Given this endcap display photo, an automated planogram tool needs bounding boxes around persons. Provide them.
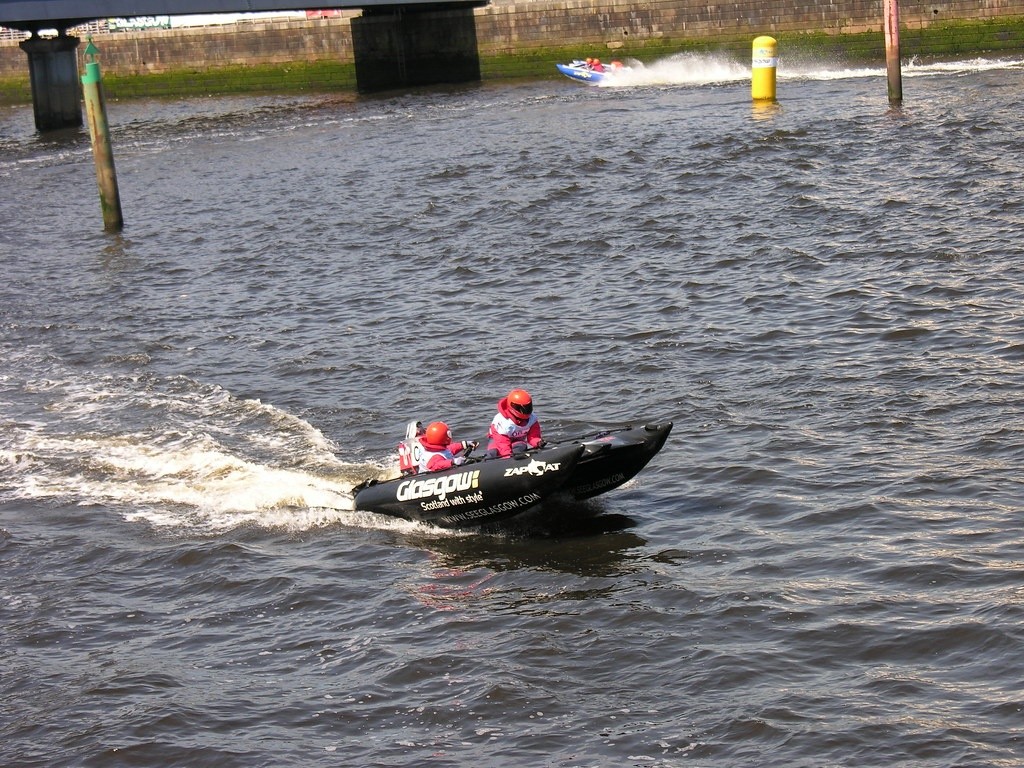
[487,389,544,460]
[585,58,605,72]
[418,421,479,474]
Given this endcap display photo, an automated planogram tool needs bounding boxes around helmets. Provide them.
[508,390,532,419]
[426,422,452,445]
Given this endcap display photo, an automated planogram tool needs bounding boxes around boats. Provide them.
[556,59,632,87]
[350,422,674,529]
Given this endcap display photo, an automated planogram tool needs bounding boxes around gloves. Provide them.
[538,440,547,448]
[454,457,467,465]
[461,440,479,451]
